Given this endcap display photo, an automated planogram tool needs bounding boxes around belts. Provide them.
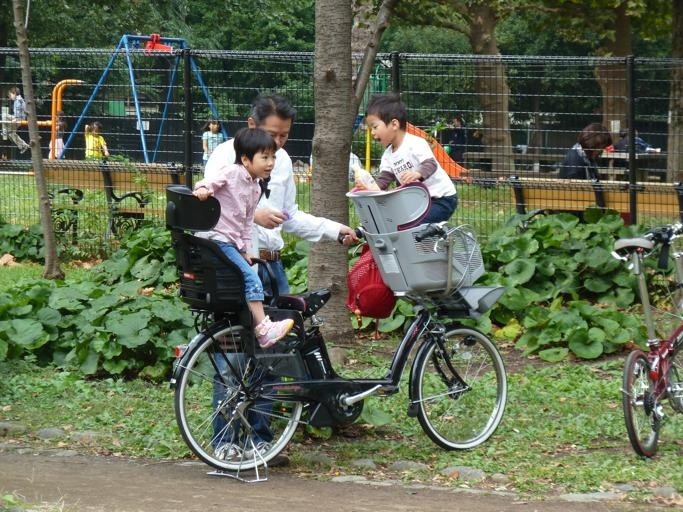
[241,249,280,261]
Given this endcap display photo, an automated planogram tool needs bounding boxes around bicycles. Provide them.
[613,183,683,457]
[166,182,507,482]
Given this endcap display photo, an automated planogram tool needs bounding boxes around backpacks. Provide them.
[346,244,399,319]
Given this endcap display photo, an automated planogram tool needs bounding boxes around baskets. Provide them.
[381,228,485,293]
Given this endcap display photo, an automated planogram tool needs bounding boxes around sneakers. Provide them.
[253,315,294,349]
[210,443,290,468]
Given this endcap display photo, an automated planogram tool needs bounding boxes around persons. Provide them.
[6,87,29,155]
[190,128,295,350]
[559,122,613,181]
[351,93,458,229]
[613,127,631,170]
[200,115,225,165]
[84,122,110,162]
[47,111,68,160]
[635,130,661,168]
[205,92,359,470]
[449,115,480,162]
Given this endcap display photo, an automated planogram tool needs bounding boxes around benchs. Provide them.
[511,176,683,229]
[33,158,186,246]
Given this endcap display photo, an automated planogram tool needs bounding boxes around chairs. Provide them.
[165,184,282,314]
[102,160,152,241]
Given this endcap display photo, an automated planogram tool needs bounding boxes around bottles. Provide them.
[351,162,382,192]
